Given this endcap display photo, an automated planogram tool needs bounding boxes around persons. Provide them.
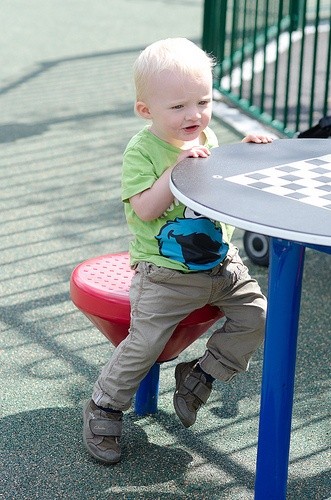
[82,37,273,461]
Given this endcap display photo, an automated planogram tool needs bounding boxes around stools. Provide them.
[71,253,225,414]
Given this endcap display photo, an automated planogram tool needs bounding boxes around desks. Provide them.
[168,138,331,500]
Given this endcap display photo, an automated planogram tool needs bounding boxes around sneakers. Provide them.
[174,361,211,428]
[83,398,123,462]
